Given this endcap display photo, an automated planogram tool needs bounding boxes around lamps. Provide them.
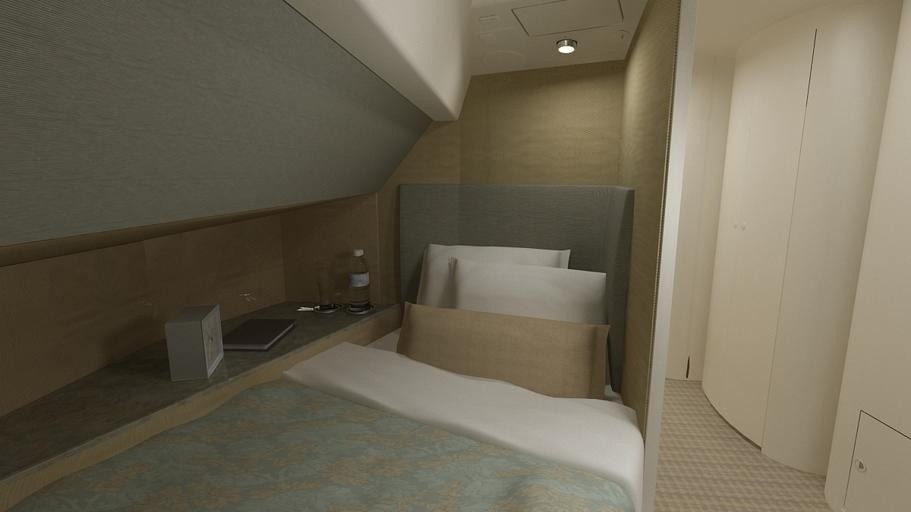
[556,36,581,59]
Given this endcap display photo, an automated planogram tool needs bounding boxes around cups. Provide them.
[314,257,337,310]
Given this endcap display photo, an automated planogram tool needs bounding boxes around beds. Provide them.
[6,244,642,512]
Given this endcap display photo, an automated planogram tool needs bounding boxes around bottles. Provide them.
[348,247,370,313]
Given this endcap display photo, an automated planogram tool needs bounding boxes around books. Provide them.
[221,316,298,353]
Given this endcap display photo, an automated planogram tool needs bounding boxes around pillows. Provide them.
[393,244,614,399]
